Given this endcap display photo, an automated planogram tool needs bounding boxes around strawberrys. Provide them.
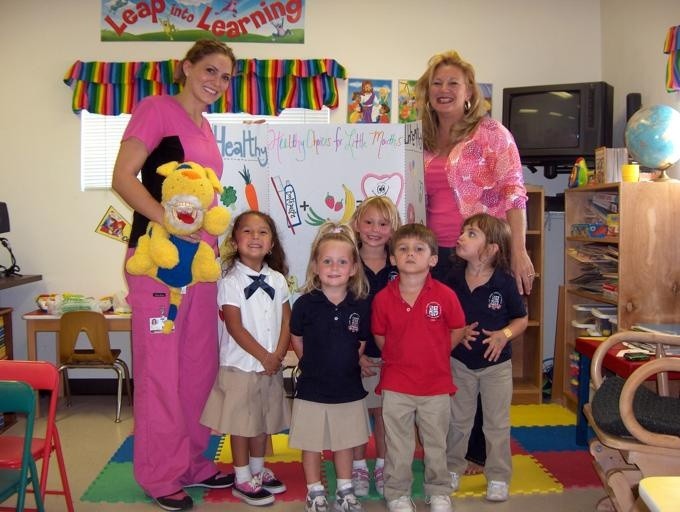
[325,192,334,209]
[335,199,343,211]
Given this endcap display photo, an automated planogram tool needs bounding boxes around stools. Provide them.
[583,326,679,512]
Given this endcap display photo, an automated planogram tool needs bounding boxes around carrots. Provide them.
[238,164,259,212]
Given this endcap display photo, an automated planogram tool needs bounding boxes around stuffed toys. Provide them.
[126,160,232,334]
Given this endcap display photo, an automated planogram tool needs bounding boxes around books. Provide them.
[567,146,679,402]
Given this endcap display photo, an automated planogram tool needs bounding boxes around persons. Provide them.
[409,51,537,476]
[434,213,529,501]
[112,38,237,512]
[198,209,293,507]
[290,222,372,512]
[369,223,467,511]
[344,195,401,499]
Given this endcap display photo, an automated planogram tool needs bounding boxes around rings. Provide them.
[528,273,533,277]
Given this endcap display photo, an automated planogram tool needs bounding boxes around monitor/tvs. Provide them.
[502,81,614,165]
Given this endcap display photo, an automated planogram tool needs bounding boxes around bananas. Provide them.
[339,184,356,225]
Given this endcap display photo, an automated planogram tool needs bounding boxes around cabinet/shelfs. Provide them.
[508,186,544,407]
[0,306,13,360]
[562,179,679,418]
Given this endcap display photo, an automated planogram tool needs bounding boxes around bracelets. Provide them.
[501,327,513,342]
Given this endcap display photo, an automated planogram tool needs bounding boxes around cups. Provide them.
[621,164,639,183]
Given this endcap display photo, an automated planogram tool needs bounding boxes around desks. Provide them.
[0,272,43,292]
[22,308,134,417]
[573,335,679,448]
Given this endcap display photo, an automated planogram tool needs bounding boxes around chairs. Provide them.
[0,358,75,512]
[49,310,133,424]
[0,380,46,512]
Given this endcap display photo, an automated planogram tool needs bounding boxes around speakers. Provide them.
[627,93,642,122]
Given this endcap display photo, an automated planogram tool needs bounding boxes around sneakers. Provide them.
[374,465,384,496]
[487,481,508,501]
[232,476,275,505]
[184,471,234,488]
[386,496,413,512]
[306,485,329,512]
[252,471,286,493]
[352,467,369,497]
[335,483,368,512]
[144,489,193,511]
[430,496,453,512]
[449,471,459,492]
